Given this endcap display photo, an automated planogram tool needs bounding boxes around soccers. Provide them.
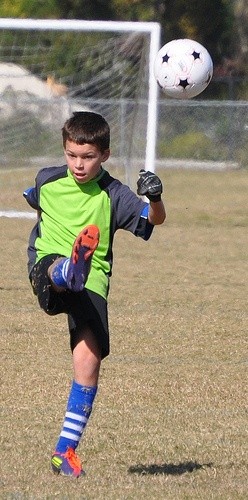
[152,37,214,102]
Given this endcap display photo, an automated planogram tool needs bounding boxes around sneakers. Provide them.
[67,224,100,291]
[51,444,87,476]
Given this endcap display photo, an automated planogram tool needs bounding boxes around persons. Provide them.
[23,111,165,475]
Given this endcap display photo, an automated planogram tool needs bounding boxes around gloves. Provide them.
[137,168,162,202]
